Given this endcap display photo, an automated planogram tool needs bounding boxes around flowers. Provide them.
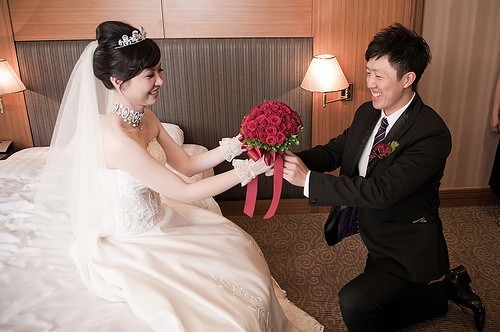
[239,100,304,152]
[368,141,400,159]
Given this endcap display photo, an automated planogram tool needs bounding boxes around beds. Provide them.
[0,147,223,332]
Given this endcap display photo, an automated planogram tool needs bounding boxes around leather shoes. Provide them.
[446,265,484,332]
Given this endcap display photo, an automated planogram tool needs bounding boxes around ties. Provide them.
[355,117,388,232]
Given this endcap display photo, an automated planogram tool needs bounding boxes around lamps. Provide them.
[0,58,27,115]
[299,53,353,108]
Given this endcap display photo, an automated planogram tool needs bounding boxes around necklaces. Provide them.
[110,103,146,130]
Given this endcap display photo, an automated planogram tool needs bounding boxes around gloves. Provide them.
[218,133,247,162]
[232,154,275,187]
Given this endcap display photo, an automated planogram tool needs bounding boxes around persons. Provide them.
[265,24,485,332]
[0,21,324,332]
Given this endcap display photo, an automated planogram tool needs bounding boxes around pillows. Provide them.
[162,122,185,149]
